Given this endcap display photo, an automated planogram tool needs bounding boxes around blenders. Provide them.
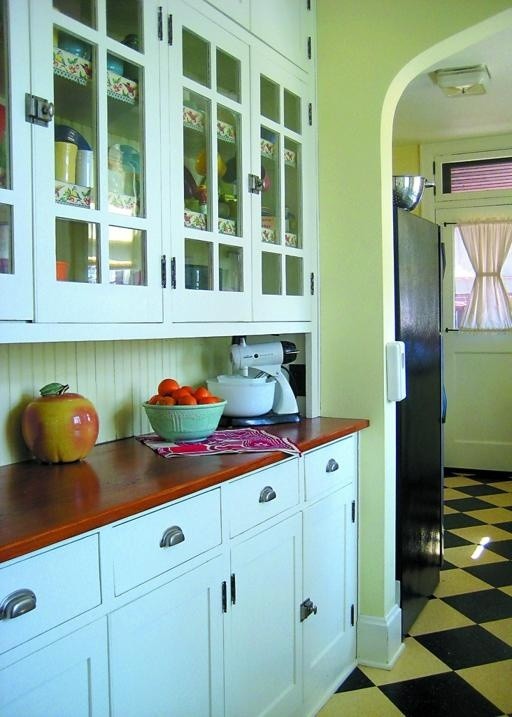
[205,337,302,428]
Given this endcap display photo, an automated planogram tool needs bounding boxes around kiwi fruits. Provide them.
[218,201,231,218]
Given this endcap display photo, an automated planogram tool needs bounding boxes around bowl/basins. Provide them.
[392,176,426,212]
[141,397,228,442]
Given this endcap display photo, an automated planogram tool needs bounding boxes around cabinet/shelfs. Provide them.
[164,2,320,340]
[0,1,164,344]
[1,480,229,715]
[221,430,363,715]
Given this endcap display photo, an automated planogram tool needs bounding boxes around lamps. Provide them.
[426,65,494,101]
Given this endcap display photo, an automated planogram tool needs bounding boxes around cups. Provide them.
[75,149,95,189]
[194,147,272,192]
[54,141,79,186]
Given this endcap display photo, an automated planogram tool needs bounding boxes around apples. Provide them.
[20,383,100,463]
[5,394,34,461]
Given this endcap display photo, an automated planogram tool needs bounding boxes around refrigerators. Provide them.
[390,207,448,643]
